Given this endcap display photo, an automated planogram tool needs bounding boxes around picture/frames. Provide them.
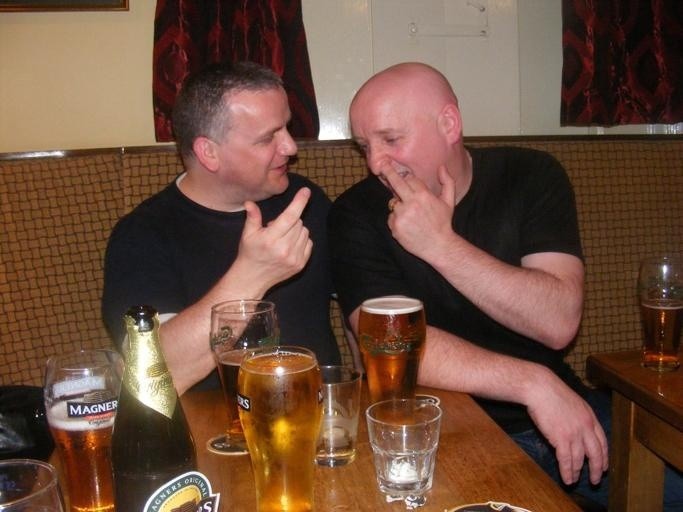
[0,0,130,14]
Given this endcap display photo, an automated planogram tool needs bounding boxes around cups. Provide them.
[43,349,118,511]
[312,366,360,465]
[358,296,427,427]
[238,345,323,511]
[365,397,441,499]
[0,458,65,511]
[635,256,682,373]
[209,300,280,441]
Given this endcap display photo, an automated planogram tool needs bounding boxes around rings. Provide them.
[391,200,399,211]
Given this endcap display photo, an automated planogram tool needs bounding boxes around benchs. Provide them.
[0,126,681,510]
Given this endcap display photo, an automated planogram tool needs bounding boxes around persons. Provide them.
[100,60,365,397]
[326,61,683,512]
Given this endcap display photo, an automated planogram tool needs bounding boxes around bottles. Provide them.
[108,307,199,511]
